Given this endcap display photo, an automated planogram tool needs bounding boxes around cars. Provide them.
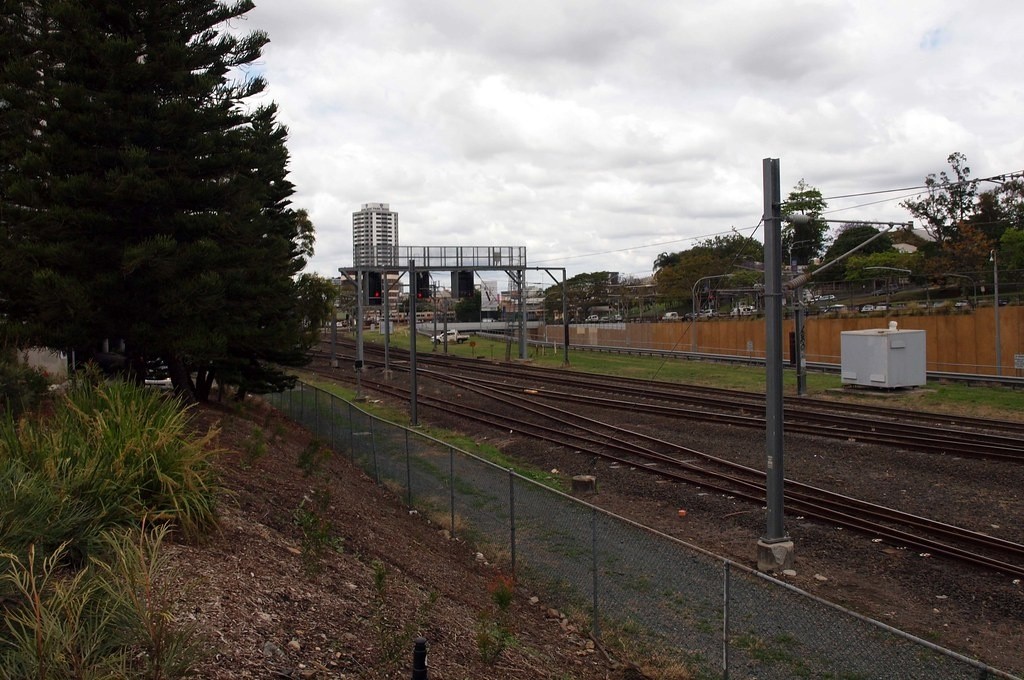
[955,299,973,309]
[570,314,623,323]
[662,306,757,320]
[860,301,944,312]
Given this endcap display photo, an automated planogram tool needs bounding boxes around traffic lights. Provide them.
[369,271,381,305]
[417,270,429,298]
[459,272,473,297]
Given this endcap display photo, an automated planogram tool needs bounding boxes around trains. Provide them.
[381,309,564,323]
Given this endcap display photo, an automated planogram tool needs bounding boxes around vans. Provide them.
[824,304,847,313]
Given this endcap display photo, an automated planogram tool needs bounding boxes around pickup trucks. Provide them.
[431,329,470,345]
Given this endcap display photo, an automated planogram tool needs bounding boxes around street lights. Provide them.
[543,292,559,343]
[692,273,734,352]
[990,249,1002,376]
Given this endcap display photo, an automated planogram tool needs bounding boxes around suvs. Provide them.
[872,283,899,297]
